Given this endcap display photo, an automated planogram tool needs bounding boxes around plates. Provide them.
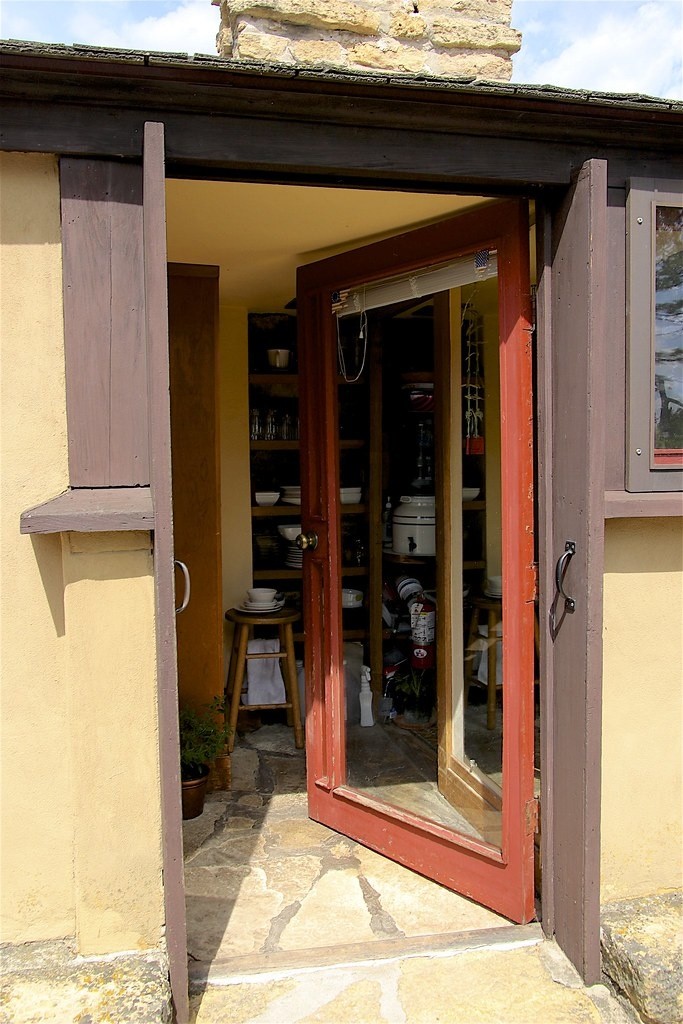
[280,485,301,505]
[484,585,502,599]
[284,543,302,568]
[234,599,281,613]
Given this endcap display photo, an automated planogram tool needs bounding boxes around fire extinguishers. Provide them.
[410,589,438,671]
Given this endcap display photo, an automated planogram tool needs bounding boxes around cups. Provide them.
[267,349,289,368]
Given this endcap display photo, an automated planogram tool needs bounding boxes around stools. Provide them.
[224,609,304,752]
[464,596,540,731]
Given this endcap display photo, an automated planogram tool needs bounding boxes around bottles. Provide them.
[248,405,299,441]
[287,660,305,727]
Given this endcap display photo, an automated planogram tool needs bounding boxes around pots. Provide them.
[393,495,436,556]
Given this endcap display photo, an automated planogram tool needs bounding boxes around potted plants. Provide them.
[179,695,234,821]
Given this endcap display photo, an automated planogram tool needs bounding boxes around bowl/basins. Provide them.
[278,525,301,541]
[339,487,361,504]
[462,487,480,501]
[251,491,280,507]
[487,575,501,588]
[247,588,277,602]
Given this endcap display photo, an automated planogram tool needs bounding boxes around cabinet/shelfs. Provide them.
[248,313,486,724]
